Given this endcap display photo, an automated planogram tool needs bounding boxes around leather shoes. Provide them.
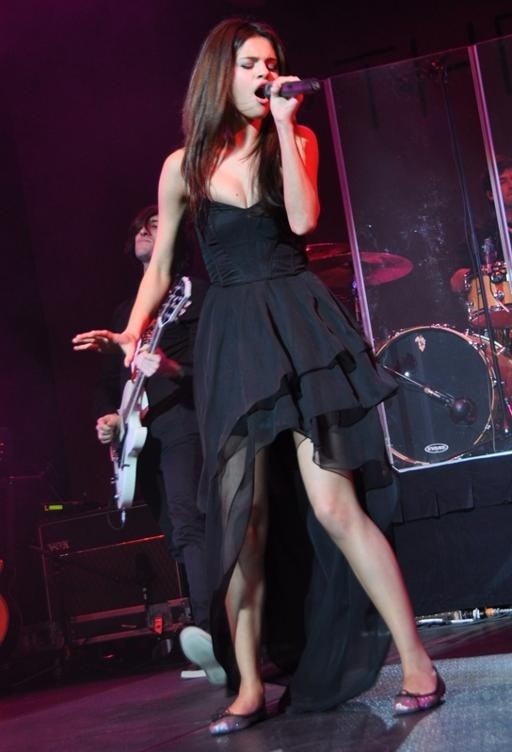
[393,666,445,715]
[209,698,265,734]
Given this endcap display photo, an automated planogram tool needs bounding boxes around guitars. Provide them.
[109,277,192,509]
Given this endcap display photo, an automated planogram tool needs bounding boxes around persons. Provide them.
[69,205,248,693]
[64,15,447,738]
[455,156,512,279]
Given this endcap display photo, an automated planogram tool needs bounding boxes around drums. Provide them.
[461,260,512,331]
[375,325,512,466]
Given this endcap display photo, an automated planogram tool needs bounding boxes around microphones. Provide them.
[447,393,480,425]
[262,75,324,100]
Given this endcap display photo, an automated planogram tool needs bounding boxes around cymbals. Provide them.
[303,249,411,289]
